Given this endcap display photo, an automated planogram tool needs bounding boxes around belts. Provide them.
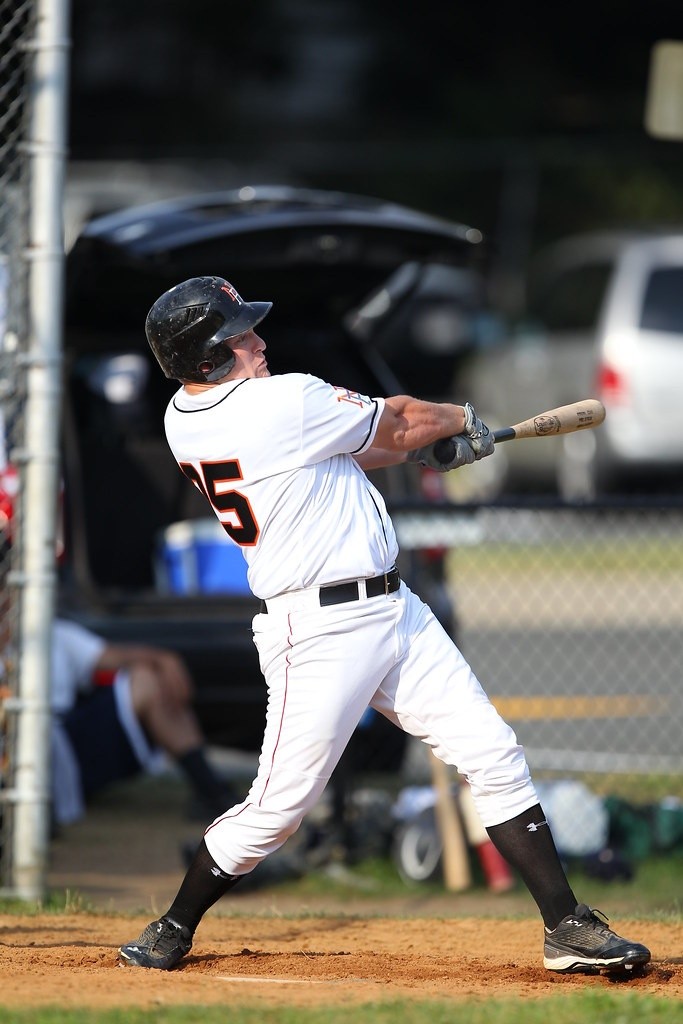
[260,566,401,614]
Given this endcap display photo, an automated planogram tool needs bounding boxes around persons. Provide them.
[118,276,652,973]
[49,620,244,825]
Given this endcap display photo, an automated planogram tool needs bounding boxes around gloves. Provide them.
[458,402,495,461]
[405,434,475,472]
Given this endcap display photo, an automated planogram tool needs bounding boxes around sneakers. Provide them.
[118,917,192,970]
[543,904,651,976]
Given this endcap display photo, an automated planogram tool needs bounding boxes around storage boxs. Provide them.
[161,520,253,594]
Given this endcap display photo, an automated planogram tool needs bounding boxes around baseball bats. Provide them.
[432,398,606,463]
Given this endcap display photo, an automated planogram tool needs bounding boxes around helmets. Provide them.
[144,277,273,383]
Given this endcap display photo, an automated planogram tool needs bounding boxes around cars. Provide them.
[444,232,683,511]
[59,183,484,775]
